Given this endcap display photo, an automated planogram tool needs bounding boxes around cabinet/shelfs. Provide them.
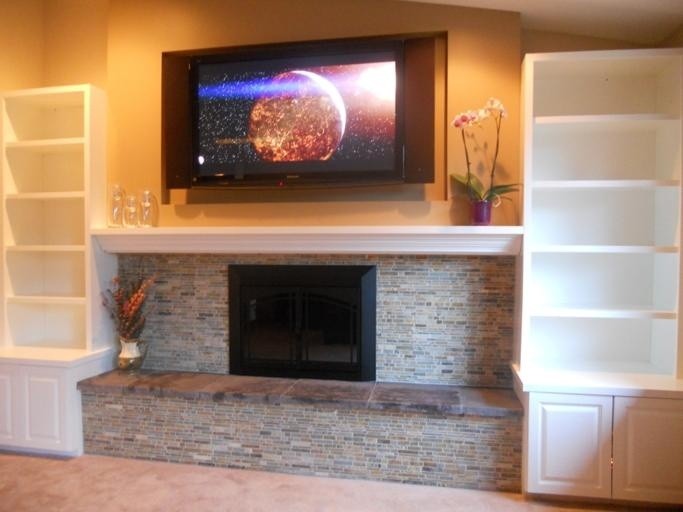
[1,84,111,458]
[507,49,683,506]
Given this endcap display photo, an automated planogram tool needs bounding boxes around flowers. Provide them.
[101,273,160,341]
[448,97,521,205]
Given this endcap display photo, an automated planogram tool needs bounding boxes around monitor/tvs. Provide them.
[186,33,405,189]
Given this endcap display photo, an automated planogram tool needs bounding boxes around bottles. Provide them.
[106,183,158,230]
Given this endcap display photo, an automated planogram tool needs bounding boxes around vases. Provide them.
[470,200,493,226]
[116,336,144,372]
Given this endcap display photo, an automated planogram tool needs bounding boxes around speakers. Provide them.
[404,36,436,185]
[161,49,187,190]
